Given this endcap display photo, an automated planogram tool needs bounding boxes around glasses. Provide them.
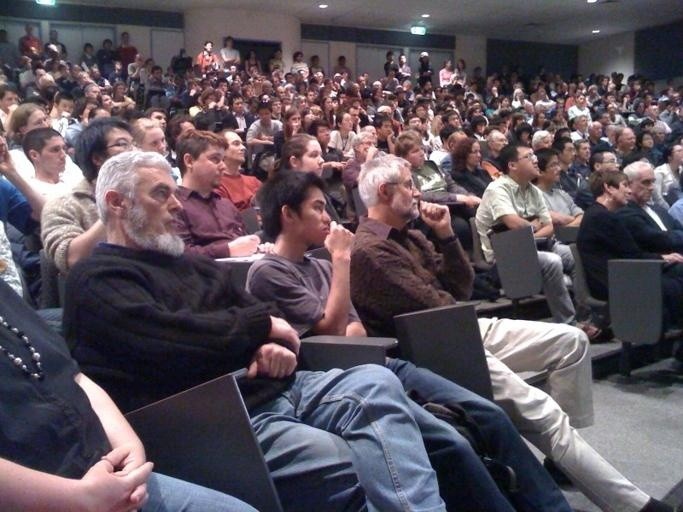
[103,141,138,152]
[387,178,414,191]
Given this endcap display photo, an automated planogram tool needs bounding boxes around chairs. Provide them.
[41,142,683,512]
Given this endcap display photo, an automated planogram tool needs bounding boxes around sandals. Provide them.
[577,322,603,341]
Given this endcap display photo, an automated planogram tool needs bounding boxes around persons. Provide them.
[0,24,683,511]
